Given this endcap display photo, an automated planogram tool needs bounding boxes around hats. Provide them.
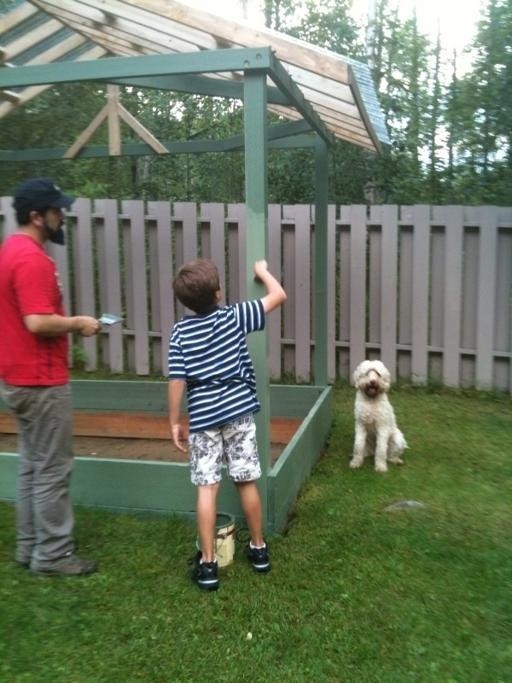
[12,179,75,210]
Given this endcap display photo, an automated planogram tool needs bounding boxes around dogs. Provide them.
[348,359,409,473]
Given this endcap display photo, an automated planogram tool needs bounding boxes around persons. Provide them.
[169,259,289,588]
[1,178,103,575]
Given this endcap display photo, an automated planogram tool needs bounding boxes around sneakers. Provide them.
[189,550,219,592]
[14,544,32,566]
[243,539,271,573]
[30,551,98,578]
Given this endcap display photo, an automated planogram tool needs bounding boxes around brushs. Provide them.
[98,313,125,326]
